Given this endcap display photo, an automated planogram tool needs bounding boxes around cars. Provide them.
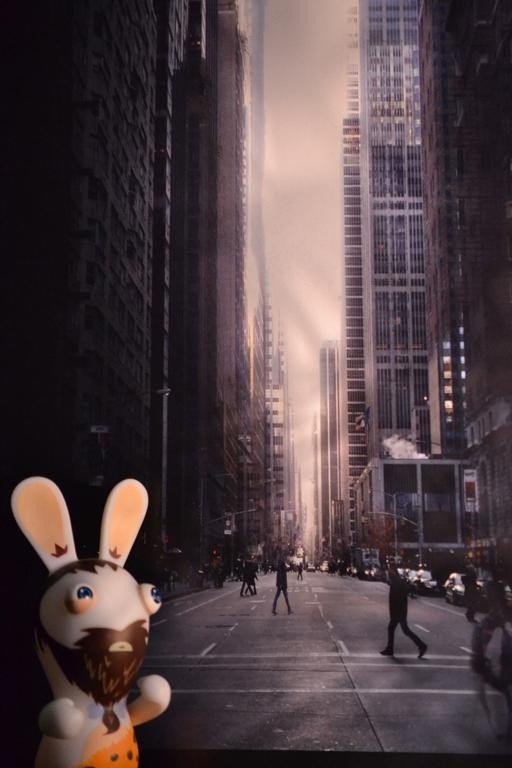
[285,556,512,614]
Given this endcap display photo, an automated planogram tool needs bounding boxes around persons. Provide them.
[240,566,258,595]
[296,561,303,581]
[272,559,292,615]
[461,565,479,624]
[380,568,427,658]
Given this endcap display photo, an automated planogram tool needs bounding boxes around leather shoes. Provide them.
[417,644,428,658]
[379,647,394,655]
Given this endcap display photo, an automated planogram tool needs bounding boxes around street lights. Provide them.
[197,477,278,589]
[362,488,423,570]
